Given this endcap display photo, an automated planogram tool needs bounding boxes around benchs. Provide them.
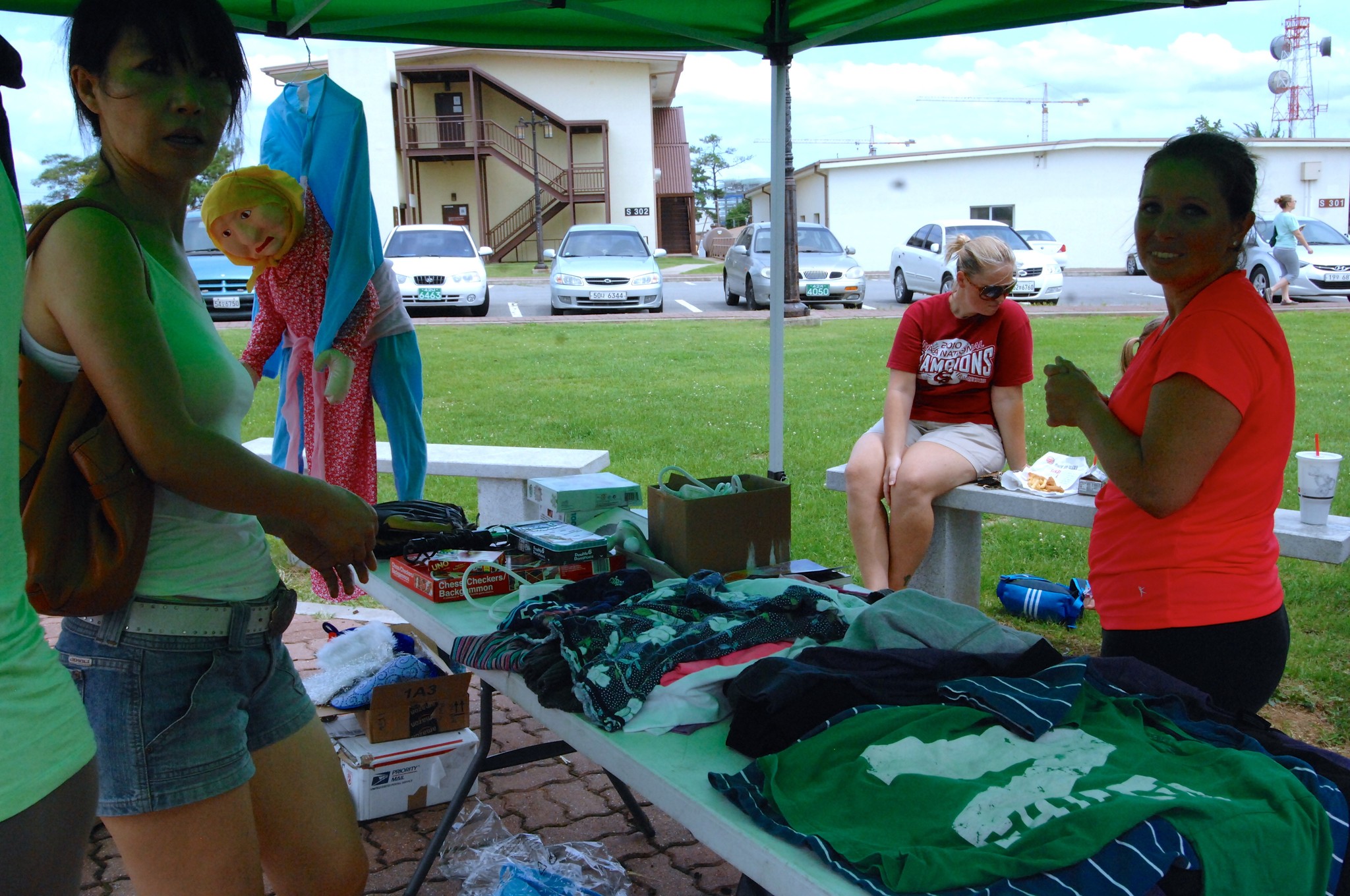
[823,463,1350,610]
[241,437,611,531]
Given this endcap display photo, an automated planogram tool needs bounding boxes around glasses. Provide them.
[962,277,1017,299]
[1287,200,1297,204]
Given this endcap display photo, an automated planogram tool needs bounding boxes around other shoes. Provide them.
[1280,299,1298,306]
[1265,288,1273,305]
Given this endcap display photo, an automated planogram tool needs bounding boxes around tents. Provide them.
[0,0,1231,479]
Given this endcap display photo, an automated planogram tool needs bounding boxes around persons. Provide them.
[0,155,98,896]
[18,0,376,896]
[1045,133,1296,714]
[844,234,1034,592]
[201,165,377,604]
[1120,317,1169,375]
[1264,194,1313,306]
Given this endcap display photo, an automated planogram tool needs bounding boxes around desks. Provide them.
[350,559,873,896]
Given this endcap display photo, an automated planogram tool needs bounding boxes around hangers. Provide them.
[288,37,327,87]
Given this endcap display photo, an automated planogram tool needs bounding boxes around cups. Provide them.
[1295,451,1343,525]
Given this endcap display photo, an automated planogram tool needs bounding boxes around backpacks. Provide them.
[998,572,1089,628]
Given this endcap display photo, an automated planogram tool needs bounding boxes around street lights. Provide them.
[514,110,554,270]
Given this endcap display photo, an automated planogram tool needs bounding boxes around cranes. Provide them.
[750,125,915,156]
[910,83,1090,142]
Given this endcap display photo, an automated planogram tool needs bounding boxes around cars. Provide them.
[181,209,255,322]
[891,219,1067,306]
[543,224,666,316]
[1127,246,1147,276]
[724,221,865,311]
[381,224,495,315]
[1237,214,1350,303]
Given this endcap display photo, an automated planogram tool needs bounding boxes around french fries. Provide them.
[1027,472,1064,493]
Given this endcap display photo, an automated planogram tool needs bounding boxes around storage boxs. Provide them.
[391,545,626,603]
[527,472,643,511]
[509,520,607,564]
[320,712,478,821]
[315,623,474,743]
[540,505,630,525]
[646,475,792,578]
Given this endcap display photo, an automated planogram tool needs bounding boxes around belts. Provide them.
[74,588,297,638]
